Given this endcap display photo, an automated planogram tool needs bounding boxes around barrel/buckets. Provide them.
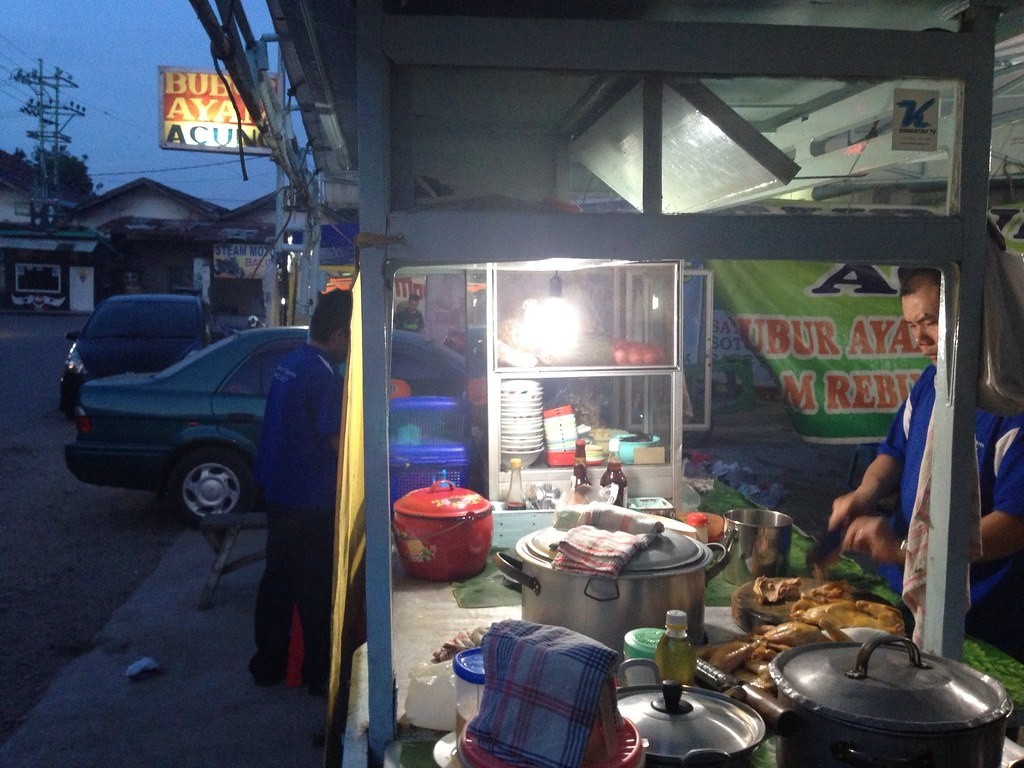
[456,712,646,768]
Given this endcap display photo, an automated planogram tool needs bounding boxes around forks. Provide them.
[527,481,563,510]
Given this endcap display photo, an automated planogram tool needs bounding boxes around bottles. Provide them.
[686,512,709,545]
[571,439,593,504]
[652,609,696,688]
[504,458,526,510]
[599,439,628,509]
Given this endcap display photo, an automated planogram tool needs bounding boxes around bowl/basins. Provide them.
[723,507,794,586]
[452,647,489,714]
[501,379,545,469]
[610,434,661,464]
[543,403,577,466]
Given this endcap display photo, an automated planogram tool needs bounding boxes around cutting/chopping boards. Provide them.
[730,576,854,635]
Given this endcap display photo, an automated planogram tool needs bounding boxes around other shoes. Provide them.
[249,654,287,684]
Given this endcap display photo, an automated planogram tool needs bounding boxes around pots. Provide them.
[492,526,732,661]
[393,480,494,580]
[769,634,1014,768]
[617,656,766,768]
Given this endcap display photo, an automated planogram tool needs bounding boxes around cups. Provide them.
[624,627,666,687]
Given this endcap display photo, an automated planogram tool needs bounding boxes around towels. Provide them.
[466,618,622,768]
[547,498,666,580]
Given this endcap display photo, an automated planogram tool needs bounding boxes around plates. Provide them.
[433,731,462,768]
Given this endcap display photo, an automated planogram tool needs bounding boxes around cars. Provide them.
[59,295,215,421]
[63,324,470,532]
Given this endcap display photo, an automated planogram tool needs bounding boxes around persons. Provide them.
[829,263,1024,643]
[394,293,424,336]
[248,288,353,696]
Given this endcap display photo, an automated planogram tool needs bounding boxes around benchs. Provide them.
[197,513,267,610]
